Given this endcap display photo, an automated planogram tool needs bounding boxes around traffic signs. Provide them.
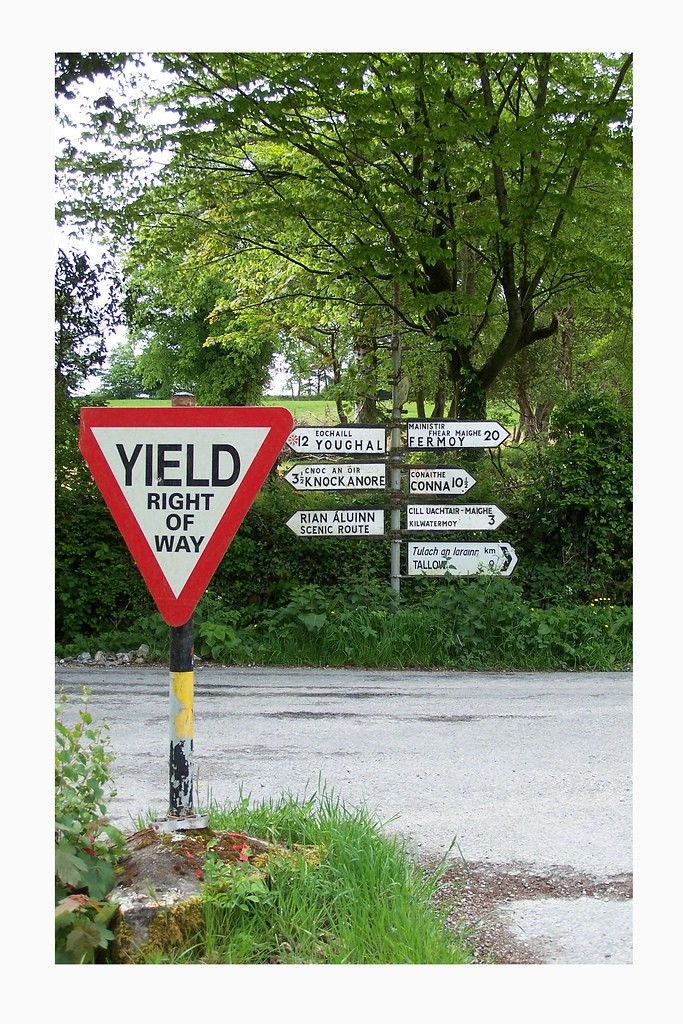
[284,426,388,456]
[408,539,518,578]
[409,465,477,497]
[283,462,386,493]
[407,417,509,449]
[406,503,509,531]
[284,509,386,537]
